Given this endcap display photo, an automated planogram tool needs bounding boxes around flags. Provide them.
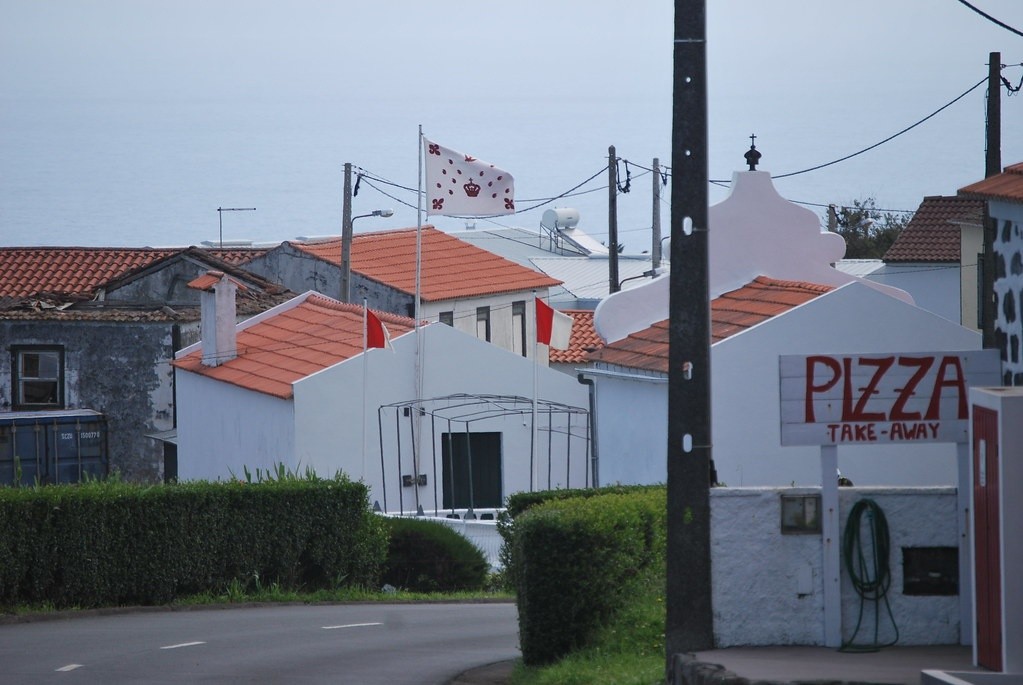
[422,134,517,217]
